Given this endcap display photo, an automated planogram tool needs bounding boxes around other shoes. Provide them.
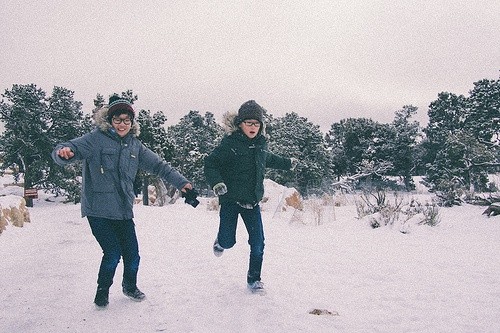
[94,286,109,310]
[247,282,265,294]
[122,286,146,302]
[213,239,224,258]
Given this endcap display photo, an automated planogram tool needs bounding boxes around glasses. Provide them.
[112,115,132,125]
[242,120,261,128]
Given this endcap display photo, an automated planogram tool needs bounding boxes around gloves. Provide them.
[290,158,299,171]
[212,182,228,197]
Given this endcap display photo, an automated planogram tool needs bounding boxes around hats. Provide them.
[108,96,135,118]
[234,100,263,128]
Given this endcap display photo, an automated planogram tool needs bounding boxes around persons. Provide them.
[52,96,193,311]
[204,100,300,295]
